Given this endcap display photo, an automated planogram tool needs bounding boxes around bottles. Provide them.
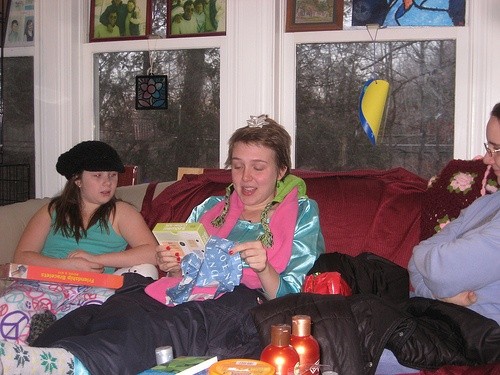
[289,315,321,375]
[260,324,300,375]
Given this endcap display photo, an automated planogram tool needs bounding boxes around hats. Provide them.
[57,141,126,179]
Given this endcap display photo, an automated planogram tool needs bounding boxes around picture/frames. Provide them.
[89,0,151,43]
[285,0,344,32]
[166,0,226,39]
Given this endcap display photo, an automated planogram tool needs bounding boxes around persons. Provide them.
[0,140,160,345]
[374,102,500,375]
[23,20,35,41]
[171,0,213,34]
[8,20,21,43]
[0,114,326,375]
[95,0,143,38]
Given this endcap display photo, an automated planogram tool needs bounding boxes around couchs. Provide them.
[0,158,500,375]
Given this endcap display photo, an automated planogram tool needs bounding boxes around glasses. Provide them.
[484,143,500,156]
